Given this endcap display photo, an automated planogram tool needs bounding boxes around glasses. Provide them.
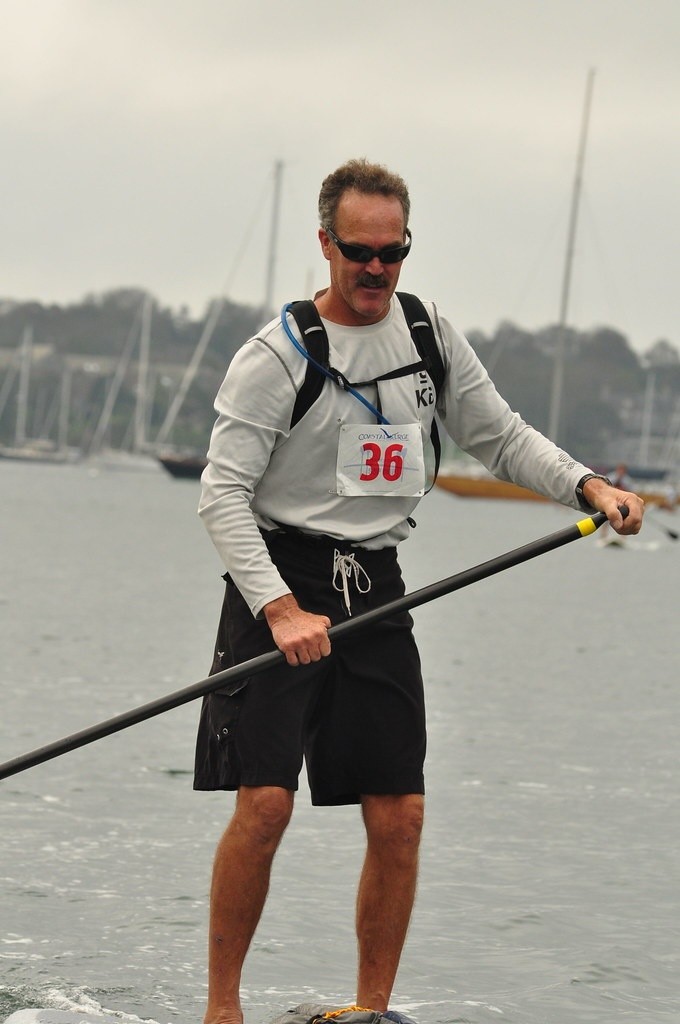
[321,223,412,264]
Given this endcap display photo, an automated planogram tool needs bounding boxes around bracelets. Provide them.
[576,474,611,514]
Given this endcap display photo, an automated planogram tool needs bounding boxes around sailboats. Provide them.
[430,70,679,509]
[0,326,70,464]
[155,158,283,481]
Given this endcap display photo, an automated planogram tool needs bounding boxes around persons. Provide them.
[193,158,644,1024]
